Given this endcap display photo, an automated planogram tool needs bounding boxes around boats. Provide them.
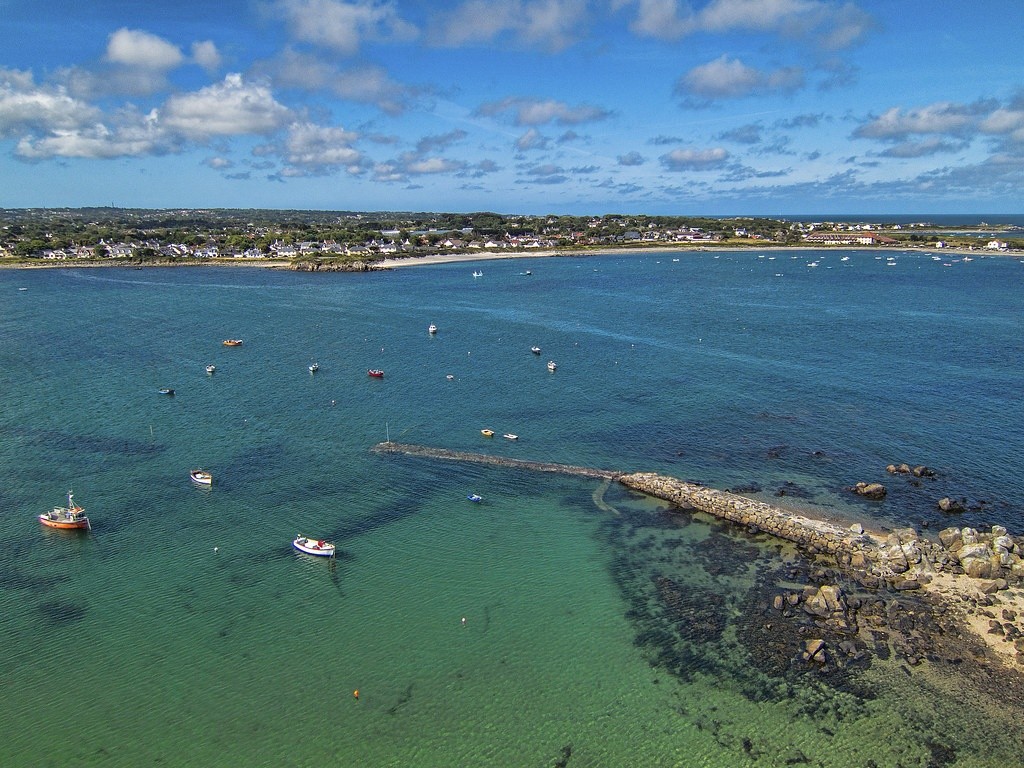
[468,494,482,503]
[548,361,556,370]
[38,491,93,531]
[531,346,541,353]
[473,271,484,277]
[190,469,212,485]
[520,271,531,276]
[447,374,453,380]
[505,434,520,440]
[158,388,176,395]
[671,247,1024,270]
[222,339,243,346]
[428,325,436,334]
[482,429,494,436]
[206,364,215,372]
[366,369,384,377]
[292,534,336,559]
[309,363,318,371]
